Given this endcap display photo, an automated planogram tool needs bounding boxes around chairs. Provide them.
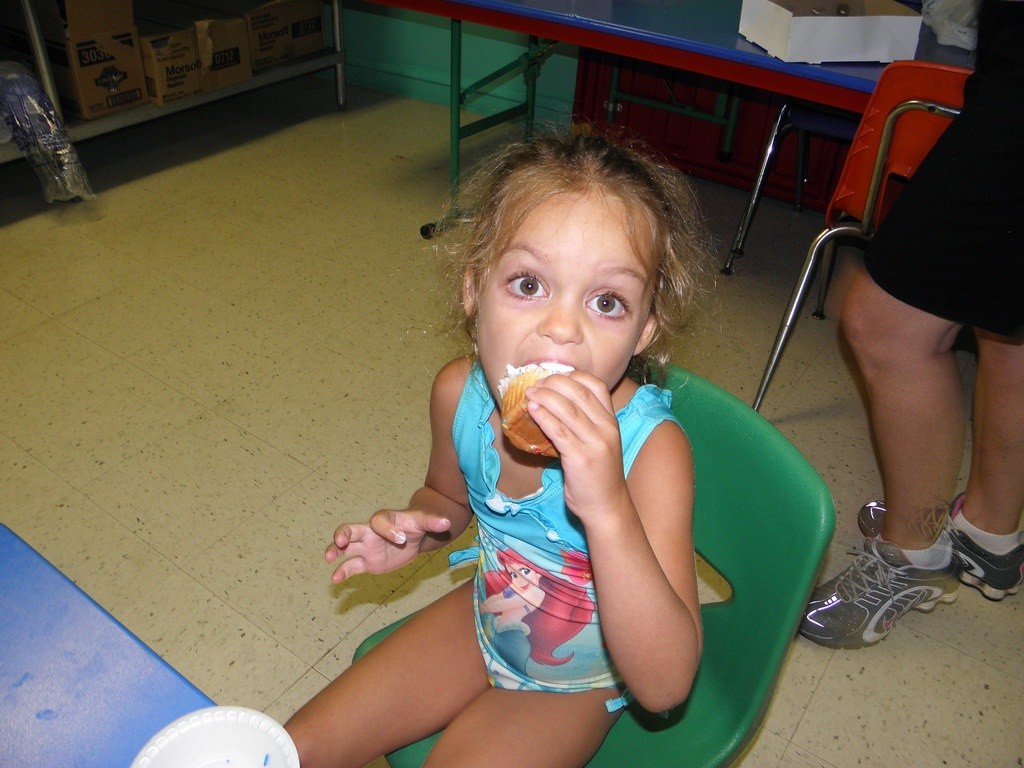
[753,61,974,416]
[353,358,837,768]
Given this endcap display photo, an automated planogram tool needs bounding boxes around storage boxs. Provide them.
[733,0,924,72]
[136,0,328,108]
[34,0,150,124]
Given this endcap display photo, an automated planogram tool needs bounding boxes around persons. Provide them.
[798,0,1023,651]
[280,127,704,767]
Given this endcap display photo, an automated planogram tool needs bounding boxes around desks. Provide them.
[402,0,975,317]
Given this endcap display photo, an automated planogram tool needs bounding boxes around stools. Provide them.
[719,99,862,277]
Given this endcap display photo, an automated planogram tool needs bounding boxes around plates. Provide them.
[130,705,301,768]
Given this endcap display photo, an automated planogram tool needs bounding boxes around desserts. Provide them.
[496,362,577,458]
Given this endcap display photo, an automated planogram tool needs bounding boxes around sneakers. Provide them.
[797,537,961,648]
[856,492,1024,601]
